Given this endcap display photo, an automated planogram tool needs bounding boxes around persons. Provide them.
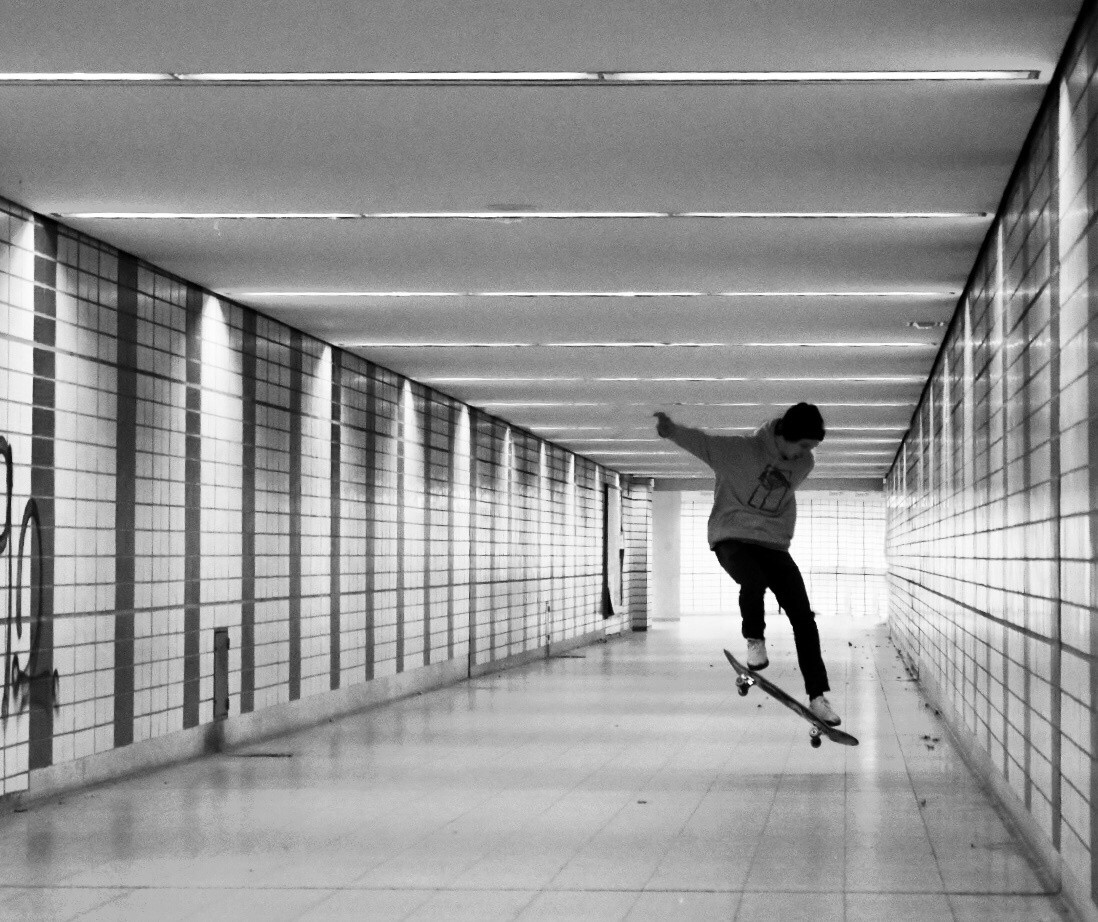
[653,402,841,725]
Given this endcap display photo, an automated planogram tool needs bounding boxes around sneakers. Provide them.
[809,696,841,726]
[747,639,768,670]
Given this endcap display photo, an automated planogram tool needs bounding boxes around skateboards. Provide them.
[724,649,860,746]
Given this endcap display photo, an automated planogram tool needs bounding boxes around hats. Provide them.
[781,403,826,441]
